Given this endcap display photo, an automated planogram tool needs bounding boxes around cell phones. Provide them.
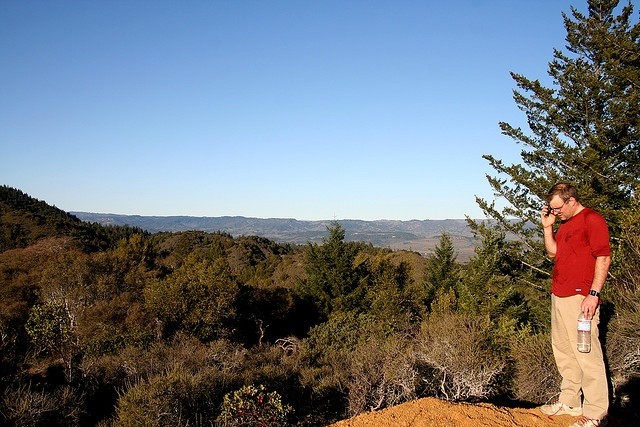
[545,206,552,217]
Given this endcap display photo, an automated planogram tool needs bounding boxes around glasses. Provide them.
[550,198,568,214]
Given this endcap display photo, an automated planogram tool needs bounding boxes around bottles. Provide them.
[576,312,592,353]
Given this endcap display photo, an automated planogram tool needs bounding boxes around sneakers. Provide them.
[571,418,602,427]
[540,402,582,420]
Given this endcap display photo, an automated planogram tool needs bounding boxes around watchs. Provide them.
[588,289,600,297]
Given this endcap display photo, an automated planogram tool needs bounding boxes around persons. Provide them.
[538,182,611,427]
